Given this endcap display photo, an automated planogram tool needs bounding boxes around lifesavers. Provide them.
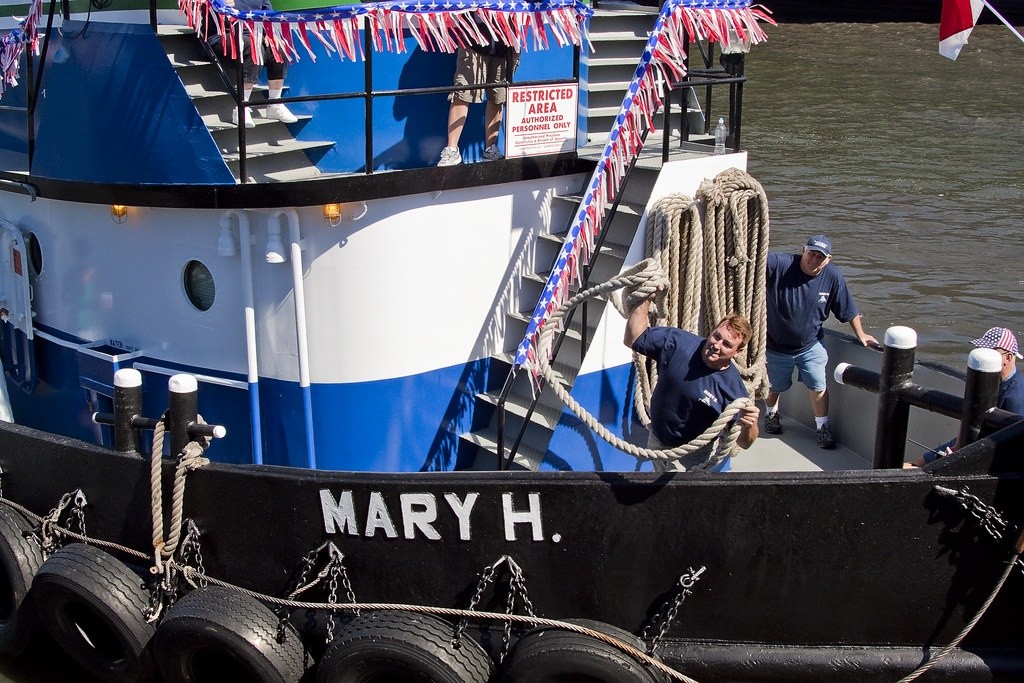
[25,540,149,681]
[134,585,309,682]
[316,611,492,681]
[497,615,666,681]
[0,501,50,616]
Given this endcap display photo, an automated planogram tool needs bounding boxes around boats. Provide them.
[1,0,1024,682]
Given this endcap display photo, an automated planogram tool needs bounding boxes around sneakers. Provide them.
[483,144,502,158]
[232,106,255,128]
[437,146,462,167]
[266,98,298,123]
[764,411,782,434]
[815,424,837,450]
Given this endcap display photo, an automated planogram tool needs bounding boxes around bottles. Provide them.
[714,117,726,155]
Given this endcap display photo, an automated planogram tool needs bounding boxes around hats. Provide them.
[968,327,1023,359]
[806,235,831,257]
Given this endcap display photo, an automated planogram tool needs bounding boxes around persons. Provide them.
[914,327,1024,467]
[437,11,521,166]
[624,283,760,473]
[763,235,880,449]
[207,0,298,128]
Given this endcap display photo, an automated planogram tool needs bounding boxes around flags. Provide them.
[939,0,985,61]
